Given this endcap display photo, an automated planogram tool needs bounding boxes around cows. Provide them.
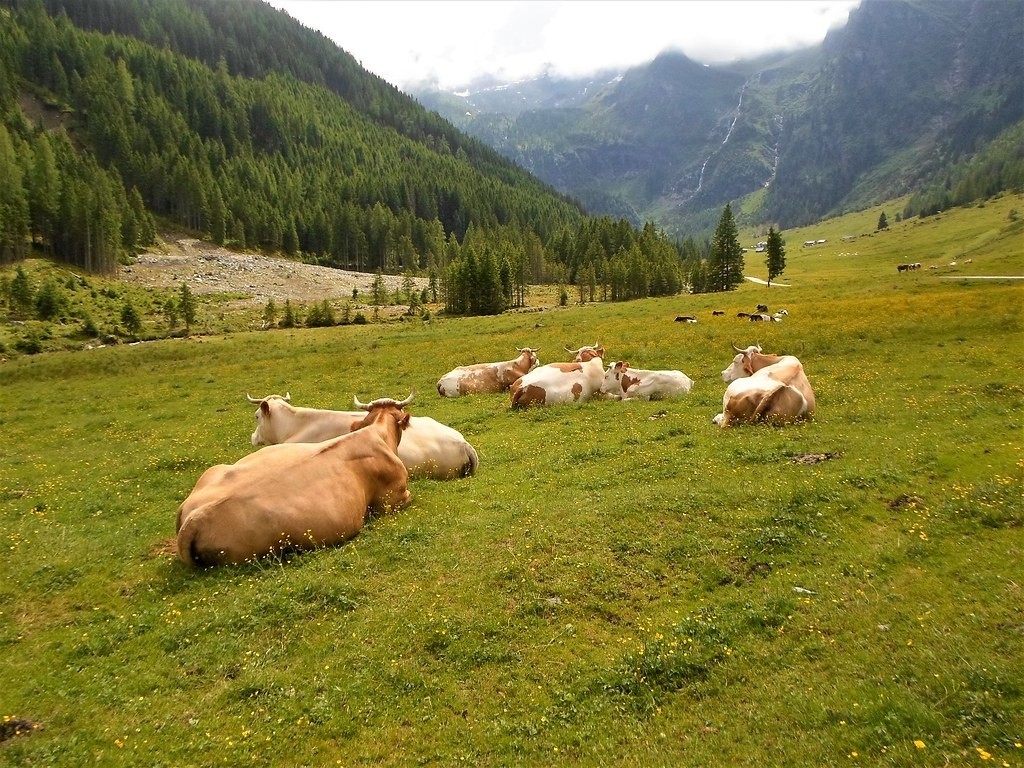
[436,341,695,414]
[897,263,921,273]
[246,392,479,480]
[148,384,414,569]
[712,339,815,428]
[674,303,788,323]
